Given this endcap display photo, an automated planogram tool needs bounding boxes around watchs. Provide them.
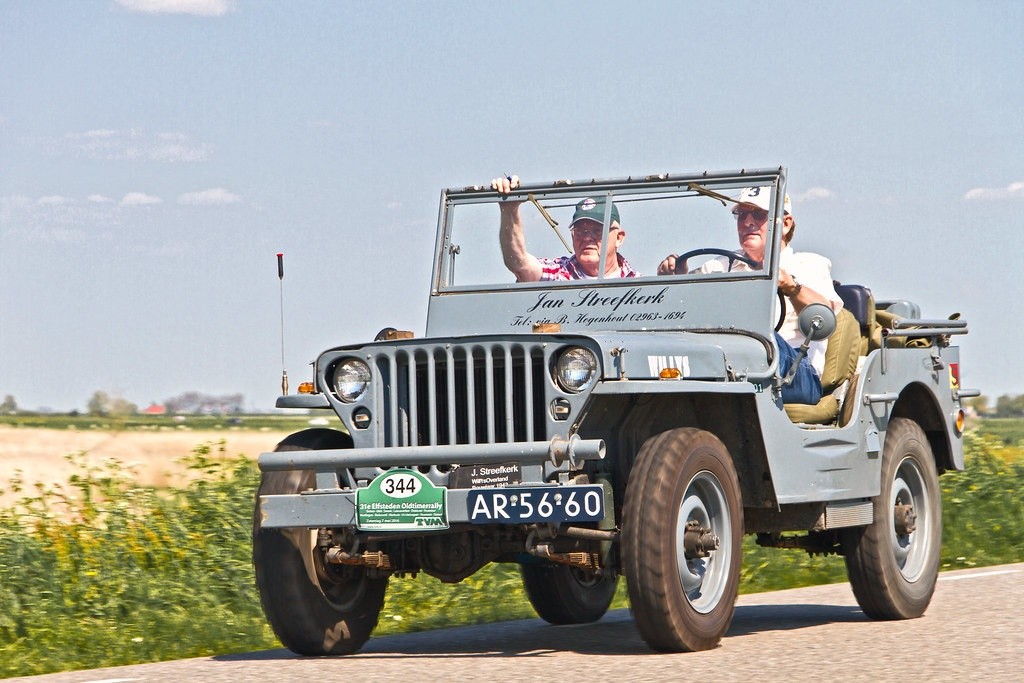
[787,275,803,300]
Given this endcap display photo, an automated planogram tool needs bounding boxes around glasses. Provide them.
[733,208,770,221]
[570,226,619,240]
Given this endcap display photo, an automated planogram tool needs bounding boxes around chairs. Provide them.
[783,285,875,427]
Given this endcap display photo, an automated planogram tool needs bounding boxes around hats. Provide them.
[567,196,620,228]
[731,186,792,215]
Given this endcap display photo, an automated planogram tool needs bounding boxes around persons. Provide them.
[490,175,643,282]
[657,187,844,407]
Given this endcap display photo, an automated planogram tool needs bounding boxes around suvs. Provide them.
[252,162,982,657]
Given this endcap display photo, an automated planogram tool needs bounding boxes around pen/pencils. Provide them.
[503,172,512,183]
